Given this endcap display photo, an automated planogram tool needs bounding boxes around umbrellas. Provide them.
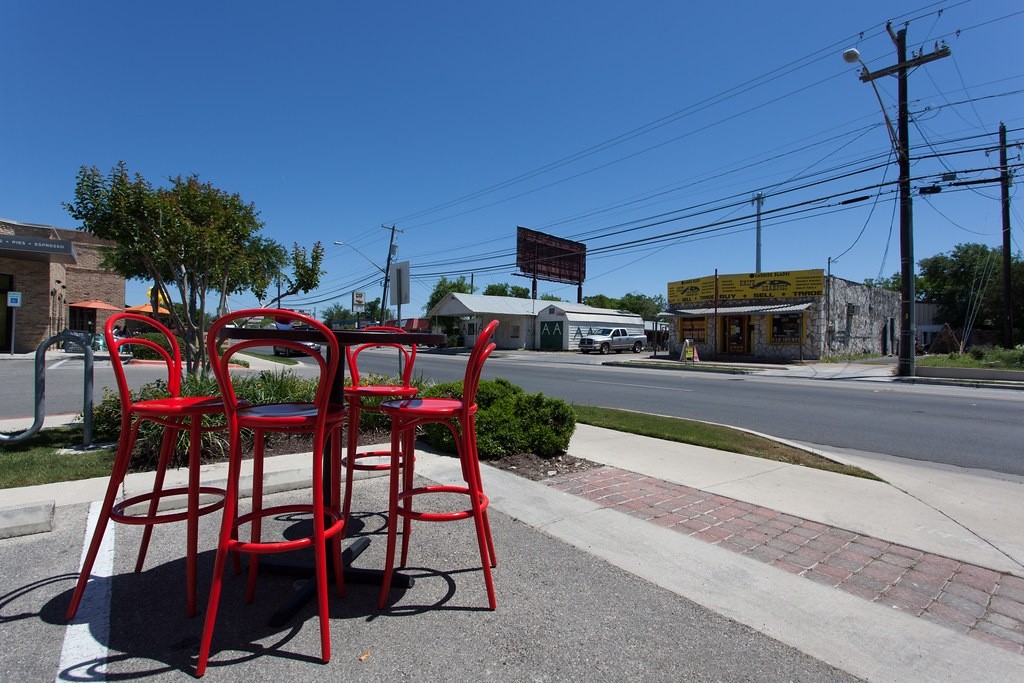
[122,303,170,329]
[64,299,122,333]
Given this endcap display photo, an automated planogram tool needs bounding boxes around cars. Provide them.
[273,341,321,358]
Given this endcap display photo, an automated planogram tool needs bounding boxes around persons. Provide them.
[111,325,131,337]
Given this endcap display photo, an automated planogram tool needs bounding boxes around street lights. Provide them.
[334,240,390,348]
[842,48,915,377]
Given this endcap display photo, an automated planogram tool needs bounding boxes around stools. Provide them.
[377,318,500,610]
[195,308,346,675]
[340,326,419,539]
[65,313,248,621]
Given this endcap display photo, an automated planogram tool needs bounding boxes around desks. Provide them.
[216,327,448,627]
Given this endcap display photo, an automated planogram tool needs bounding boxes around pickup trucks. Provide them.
[578,326,648,355]
